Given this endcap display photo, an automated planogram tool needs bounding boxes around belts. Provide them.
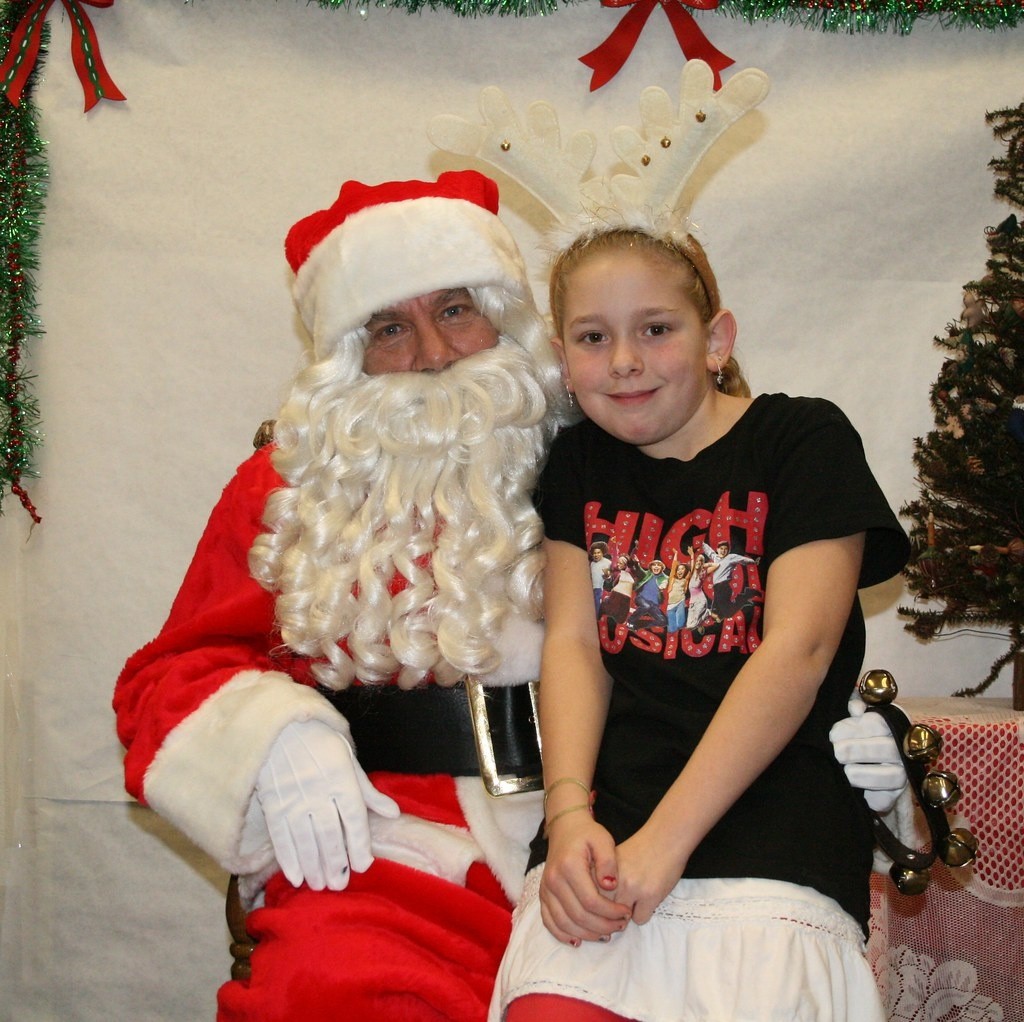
[313,682,545,797]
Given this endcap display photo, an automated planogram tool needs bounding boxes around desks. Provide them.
[849,692,1024,1021]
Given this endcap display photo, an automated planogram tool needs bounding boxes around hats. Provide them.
[284,169,518,343]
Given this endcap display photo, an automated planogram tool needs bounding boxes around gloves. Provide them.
[248,718,402,891]
[828,689,912,813]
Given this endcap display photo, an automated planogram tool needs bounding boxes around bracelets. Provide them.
[542,776,597,841]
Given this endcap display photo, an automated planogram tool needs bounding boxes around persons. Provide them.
[112,169,972,1022]
[486,223,913,1022]
